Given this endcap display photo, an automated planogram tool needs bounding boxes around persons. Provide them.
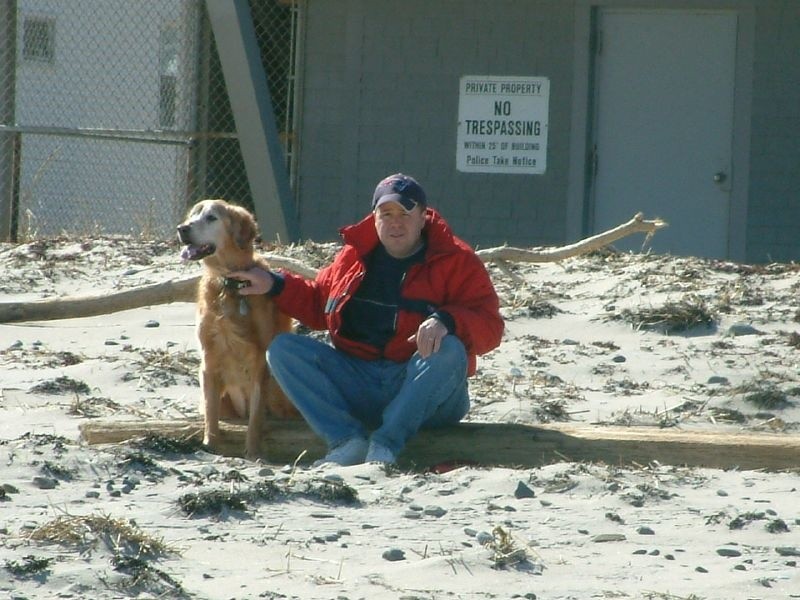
[227,173,505,475]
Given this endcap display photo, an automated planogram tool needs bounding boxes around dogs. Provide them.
[176,199,303,463]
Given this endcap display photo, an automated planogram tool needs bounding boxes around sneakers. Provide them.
[310,440,369,467]
[365,439,398,465]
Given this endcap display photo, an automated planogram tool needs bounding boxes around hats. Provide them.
[370,173,429,214]
